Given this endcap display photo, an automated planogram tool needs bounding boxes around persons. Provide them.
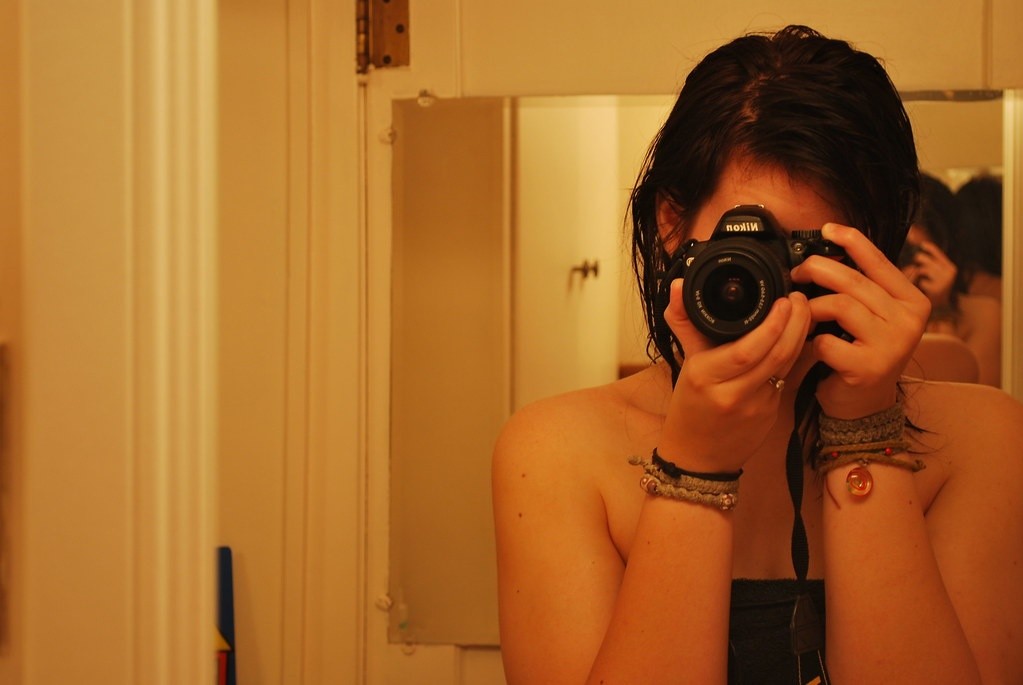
[492,25,1023,685]
[896,169,1002,388]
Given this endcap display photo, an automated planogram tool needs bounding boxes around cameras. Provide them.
[893,242,918,270]
[680,204,855,342]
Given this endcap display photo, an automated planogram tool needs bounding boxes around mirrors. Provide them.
[0,0,1023,685]
[510,93,1002,410]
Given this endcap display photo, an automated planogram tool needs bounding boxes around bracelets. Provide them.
[811,391,926,510]
[626,445,743,511]
